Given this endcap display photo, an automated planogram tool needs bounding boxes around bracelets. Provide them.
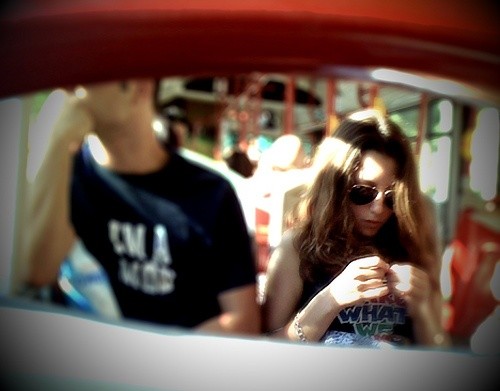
[290,310,312,345]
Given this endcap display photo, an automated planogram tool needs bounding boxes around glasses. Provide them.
[345,185,398,209]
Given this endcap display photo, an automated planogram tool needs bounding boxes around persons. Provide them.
[253,105,454,356]
[16,77,260,343]
